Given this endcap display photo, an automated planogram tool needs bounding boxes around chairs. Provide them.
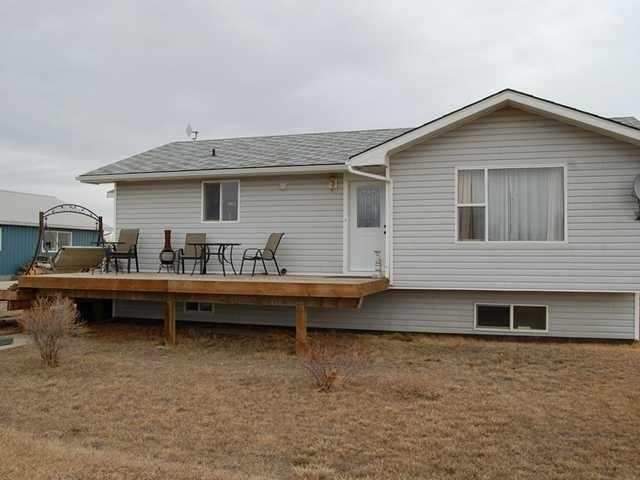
[239,231,288,276]
[105,227,144,273]
[175,232,211,275]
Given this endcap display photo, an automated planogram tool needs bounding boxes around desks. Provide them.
[184,242,242,277]
[89,241,126,275]
